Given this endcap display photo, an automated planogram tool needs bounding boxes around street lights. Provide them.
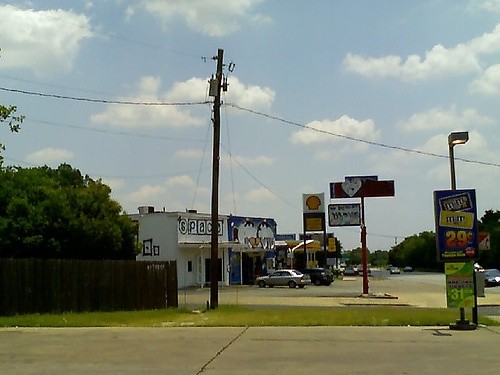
[447,131,470,324]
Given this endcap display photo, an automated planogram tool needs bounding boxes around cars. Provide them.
[300,267,335,286]
[484,268,500,286]
[403,267,412,272]
[390,267,400,274]
[386,266,392,270]
[256,269,311,288]
[340,264,371,276]
